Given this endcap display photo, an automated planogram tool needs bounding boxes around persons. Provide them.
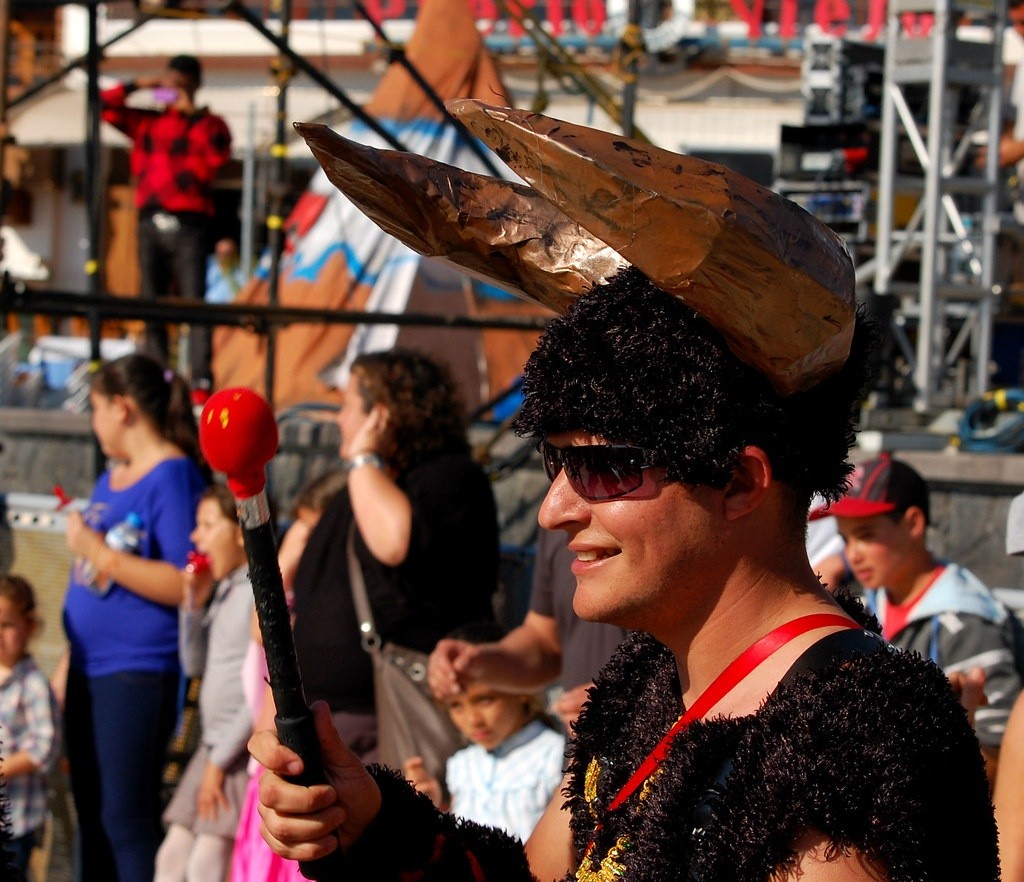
[246,98,1001,882]
[974,0,1024,226]
[102,56,231,389]
[805,453,1024,882]
[0,354,214,882]
[156,349,597,882]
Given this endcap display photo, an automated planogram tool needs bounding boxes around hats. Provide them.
[809,452,927,522]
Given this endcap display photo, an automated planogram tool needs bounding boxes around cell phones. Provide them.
[155,89,179,103]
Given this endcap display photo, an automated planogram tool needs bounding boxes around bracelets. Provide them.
[343,452,380,470]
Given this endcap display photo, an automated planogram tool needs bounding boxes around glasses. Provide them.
[541,440,663,502]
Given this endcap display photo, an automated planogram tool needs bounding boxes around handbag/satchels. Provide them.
[372,642,470,785]
